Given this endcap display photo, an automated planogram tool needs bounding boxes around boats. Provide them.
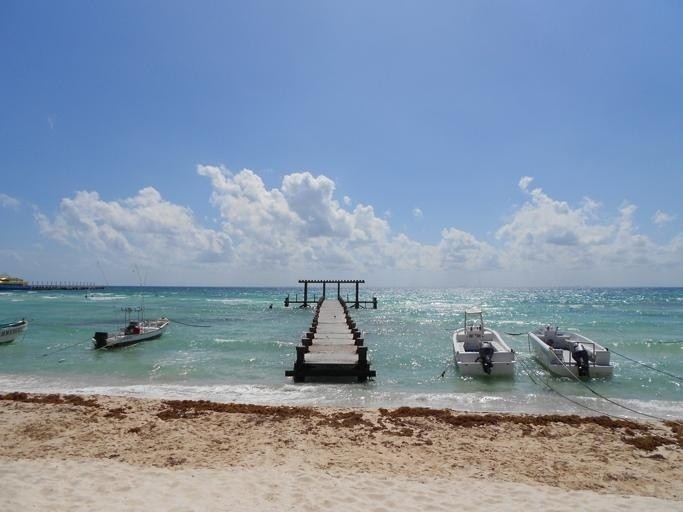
[0,317,29,345]
[452,306,517,378]
[91,305,170,351]
[526,317,616,379]
